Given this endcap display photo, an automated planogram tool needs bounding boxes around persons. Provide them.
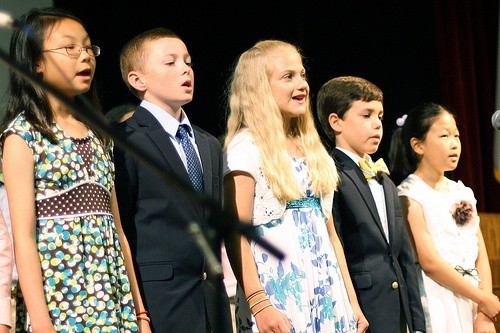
[316,76,426,333]
[227,41,368,333]
[393,101,500,333]
[0,8,239,333]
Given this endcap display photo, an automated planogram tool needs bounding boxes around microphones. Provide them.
[492,110,500,132]
[0,10,19,29]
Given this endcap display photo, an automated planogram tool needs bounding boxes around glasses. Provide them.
[41,43,101,57]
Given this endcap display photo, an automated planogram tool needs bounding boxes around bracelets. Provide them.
[137,317,150,323]
[136,310,148,315]
[246,290,272,316]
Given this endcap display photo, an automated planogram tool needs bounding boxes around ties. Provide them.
[176,124,204,191]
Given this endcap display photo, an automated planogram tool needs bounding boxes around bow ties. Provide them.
[359,153,391,182]
